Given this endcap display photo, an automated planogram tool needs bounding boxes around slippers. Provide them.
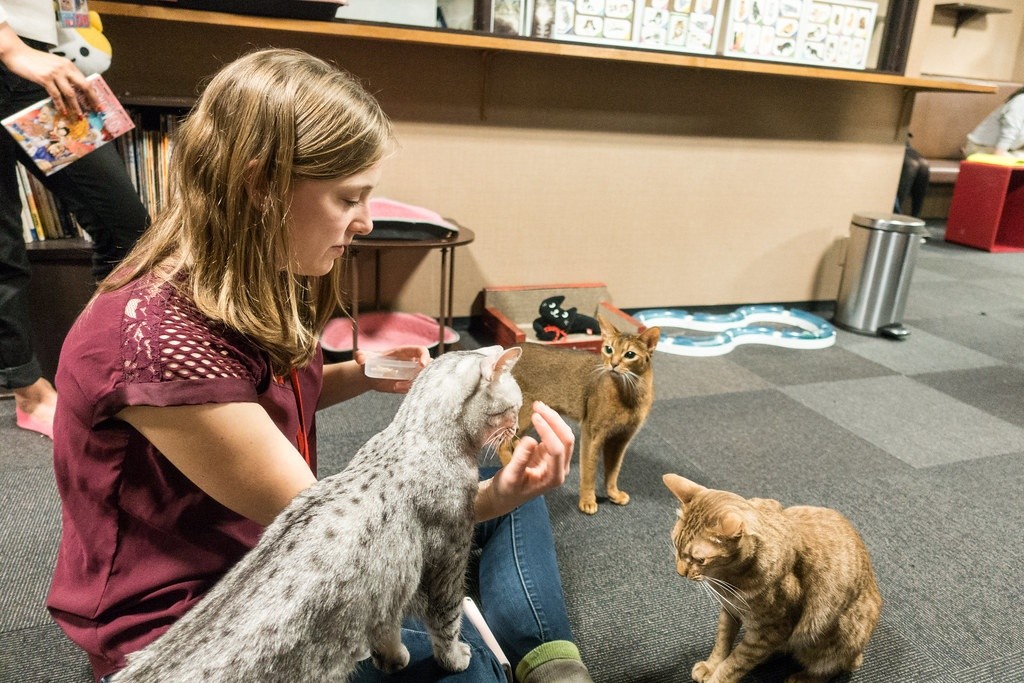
[16,401,56,442]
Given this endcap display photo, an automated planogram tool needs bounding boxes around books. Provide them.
[13,109,190,244]
[0,73,135,176]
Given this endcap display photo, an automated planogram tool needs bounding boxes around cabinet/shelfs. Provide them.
[0,95,206,386]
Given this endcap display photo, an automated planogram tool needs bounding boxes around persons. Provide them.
[0,0,152,441]
[43,50,592,683]
[964,87,1024,158]
[893,133,930,243]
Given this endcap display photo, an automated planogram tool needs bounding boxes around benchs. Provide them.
[901,72,1024,219]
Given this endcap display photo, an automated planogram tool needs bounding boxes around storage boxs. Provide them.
[478,283,647,351]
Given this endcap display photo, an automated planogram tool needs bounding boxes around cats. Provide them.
[496,313,660,515]
[107,345,523,683]
[661,472,882,683]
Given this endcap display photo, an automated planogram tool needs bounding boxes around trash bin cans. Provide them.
[830,209,927,339]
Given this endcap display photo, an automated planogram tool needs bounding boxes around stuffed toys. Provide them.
[49,10,111,75]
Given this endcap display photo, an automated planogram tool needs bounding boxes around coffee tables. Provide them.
[945,158,1024,253]
[322,220,475,362]
